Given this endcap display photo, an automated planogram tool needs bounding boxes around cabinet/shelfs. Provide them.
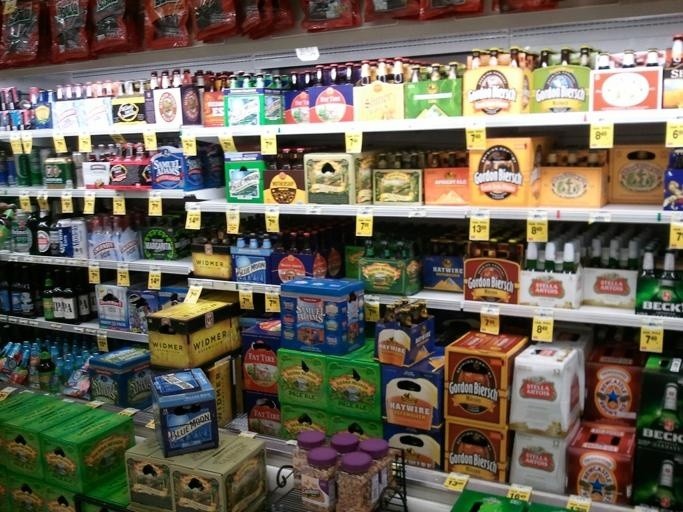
[0,108,683,512]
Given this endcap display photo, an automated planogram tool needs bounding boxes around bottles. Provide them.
[0,141,682,212]
[646,459,676,512]
[381,296,430,331]
[2,32,682,111]
[1,196,683,280]
[0,264,109,394]
[651,381,681,436]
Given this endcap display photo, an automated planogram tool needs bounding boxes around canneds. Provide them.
[56,213,145,258]
[0,147,73,188]
